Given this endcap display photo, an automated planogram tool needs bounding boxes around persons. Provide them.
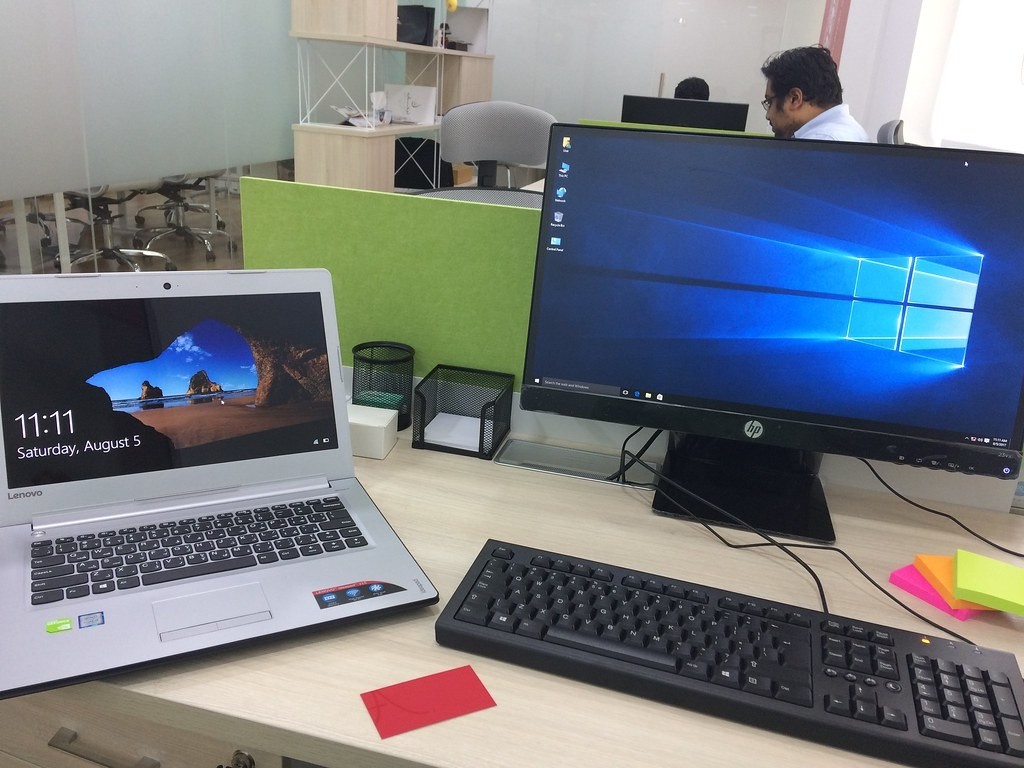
[761,44,870,144]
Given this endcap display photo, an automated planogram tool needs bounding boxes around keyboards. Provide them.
[435,538,1024,768]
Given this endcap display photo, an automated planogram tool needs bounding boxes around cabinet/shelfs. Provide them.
[290,0,497,194]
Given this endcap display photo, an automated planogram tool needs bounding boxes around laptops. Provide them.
[0,266,440,700]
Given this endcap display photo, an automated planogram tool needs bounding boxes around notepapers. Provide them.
[887,547,1024,621]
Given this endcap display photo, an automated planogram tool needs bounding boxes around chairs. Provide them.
[415,100,559,209]
[0,169,240,274]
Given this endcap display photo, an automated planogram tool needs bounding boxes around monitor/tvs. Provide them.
[621,95,750,133]
[519,121,1024,549]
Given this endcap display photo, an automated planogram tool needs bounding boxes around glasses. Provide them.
[761,87,807,112]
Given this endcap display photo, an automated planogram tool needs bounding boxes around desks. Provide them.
[0,176,1024,768]
